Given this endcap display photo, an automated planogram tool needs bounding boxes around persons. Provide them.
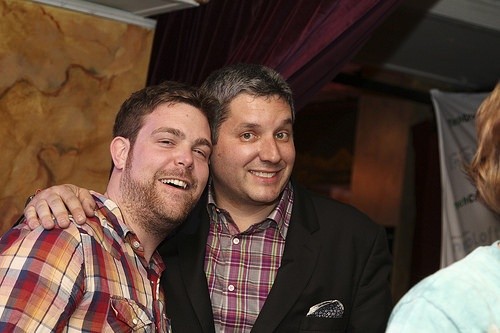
[383,84,500,333]
[22,62,392,333]
[0,79,230,333]
